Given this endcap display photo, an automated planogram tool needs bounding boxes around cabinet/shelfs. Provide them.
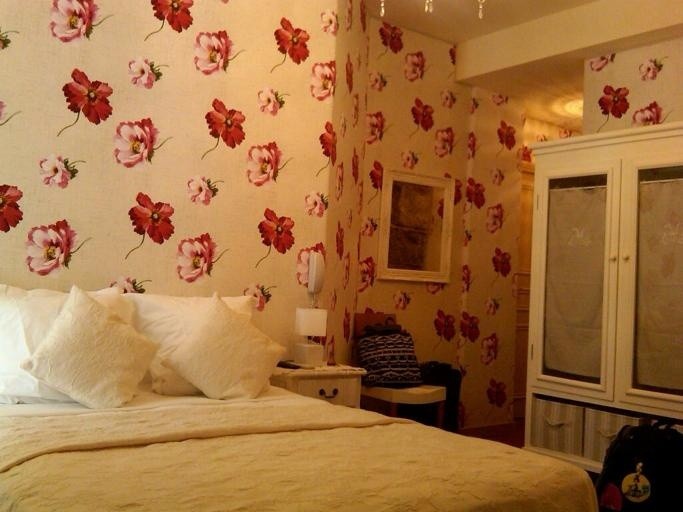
[522,120,683,475]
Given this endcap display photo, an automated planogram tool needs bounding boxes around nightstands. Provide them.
[270,363,369,408]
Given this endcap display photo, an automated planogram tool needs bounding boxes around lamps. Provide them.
[288,306,329,368]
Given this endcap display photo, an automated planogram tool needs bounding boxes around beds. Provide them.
[0,386,599,511]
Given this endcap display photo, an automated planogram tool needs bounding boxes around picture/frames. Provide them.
[374,167,458,285]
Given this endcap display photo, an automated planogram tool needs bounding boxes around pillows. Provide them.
[0,283,135,404]
[158,292,287,401]
[134,294,260,397]
[20,285,160,408]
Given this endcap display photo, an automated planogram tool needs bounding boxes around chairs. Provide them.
[354,311,449,428]
